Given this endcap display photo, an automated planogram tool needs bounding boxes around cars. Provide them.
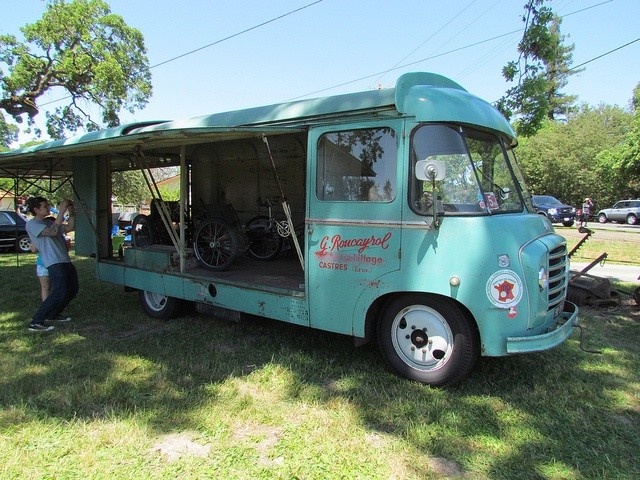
[0,211,32,253]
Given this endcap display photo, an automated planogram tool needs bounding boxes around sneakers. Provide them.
[45,316,72,321]
[29,321,55,331]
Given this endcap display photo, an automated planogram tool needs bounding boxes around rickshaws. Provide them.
[131,198,194,247]
[193,192,305,273]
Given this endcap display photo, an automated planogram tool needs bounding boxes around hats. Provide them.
[583,198,589,202]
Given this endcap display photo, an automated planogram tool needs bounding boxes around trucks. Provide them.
[0,72,579,387]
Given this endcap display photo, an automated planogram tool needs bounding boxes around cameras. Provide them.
[64,200,73,207]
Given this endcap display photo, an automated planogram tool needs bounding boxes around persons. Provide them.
[30,215,57,304]
[26,197,76,333]
[579,198,593,228]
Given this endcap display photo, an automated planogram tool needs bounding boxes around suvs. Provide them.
[597,200,640,224]
[529,194,576,227]
